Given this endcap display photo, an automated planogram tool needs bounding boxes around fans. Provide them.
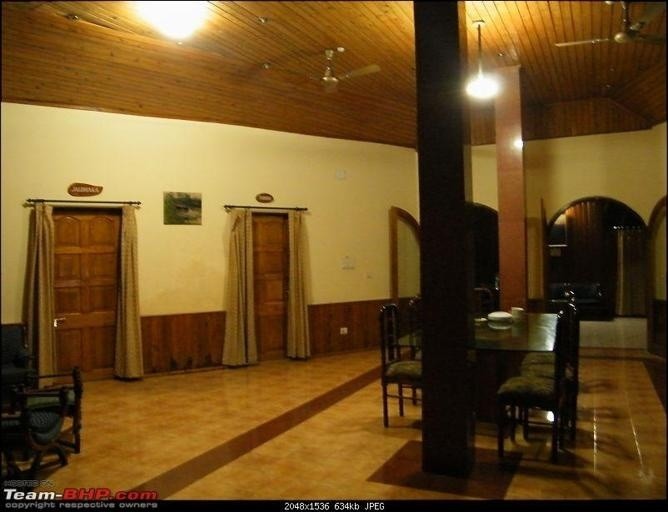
[555,1,666,47]
[310,50,379,88]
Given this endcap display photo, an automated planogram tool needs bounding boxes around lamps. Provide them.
[465,20,497,99]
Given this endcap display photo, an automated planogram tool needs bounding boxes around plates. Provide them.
[488,321,512,330]
[488,311,513,323]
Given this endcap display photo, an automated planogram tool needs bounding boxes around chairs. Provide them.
[380,286,580,465]
[1,323,82,471]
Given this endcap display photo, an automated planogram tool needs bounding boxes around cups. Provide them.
[511,307,525,324]
